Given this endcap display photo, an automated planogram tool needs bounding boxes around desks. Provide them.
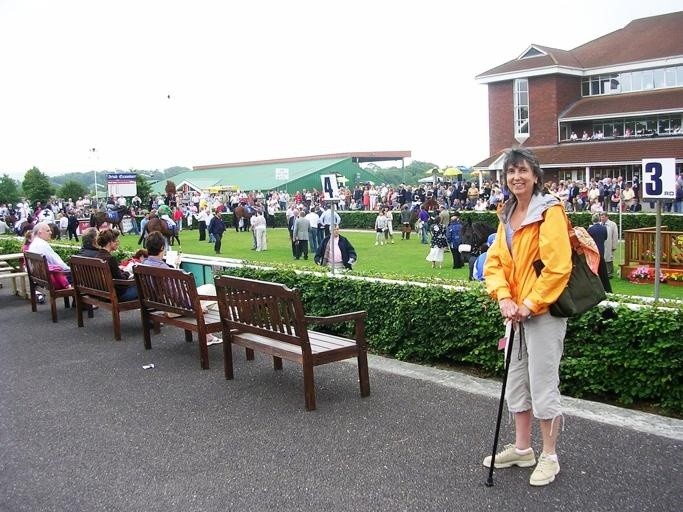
[0,253,27,296]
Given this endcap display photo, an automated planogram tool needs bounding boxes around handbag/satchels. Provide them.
[377,228,383,232]
[47,266,70,289]
[533,243,607,317]
[404,224,411,233]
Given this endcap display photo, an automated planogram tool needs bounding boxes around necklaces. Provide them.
[509,222,521,231]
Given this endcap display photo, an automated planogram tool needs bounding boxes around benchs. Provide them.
[132,264,254,369]
[0,272,29,299]
[70,254,142,341]
[0,266,27,272]
[214,274,370,411]
[24,252,74,322]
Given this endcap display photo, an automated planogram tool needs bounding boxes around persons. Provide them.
[96,229,165,329]
[482,146,573,486]
[0,178,509,283]
[568,122,681,142]
[663,175,683,213]
[23,230,46,304]
[29,222,99,310]
[542,176,641,296]
[143,231,240,345]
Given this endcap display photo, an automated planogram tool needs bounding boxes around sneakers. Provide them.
[529,454,560,486]
[483,444,536,468]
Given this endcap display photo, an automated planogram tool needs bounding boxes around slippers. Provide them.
[207,337,223,346]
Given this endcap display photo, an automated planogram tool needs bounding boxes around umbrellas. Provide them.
[425,168,443,174]
[471,170,487,177]
[455,166,469,172]
[443,168,462,184]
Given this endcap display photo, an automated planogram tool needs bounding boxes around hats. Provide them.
[487,233,496,247]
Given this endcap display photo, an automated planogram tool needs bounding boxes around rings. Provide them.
[516,320,521,323]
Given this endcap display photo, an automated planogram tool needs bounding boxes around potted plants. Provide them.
[626,235,683,286]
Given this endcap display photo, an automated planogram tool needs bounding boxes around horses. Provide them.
[89,205,132,236]
[137,217,182,246]
[407,196,439,223]
[459,215,496,281]
[13,220,61,241]
[232,206,264,232]
[414,216,435,240]
